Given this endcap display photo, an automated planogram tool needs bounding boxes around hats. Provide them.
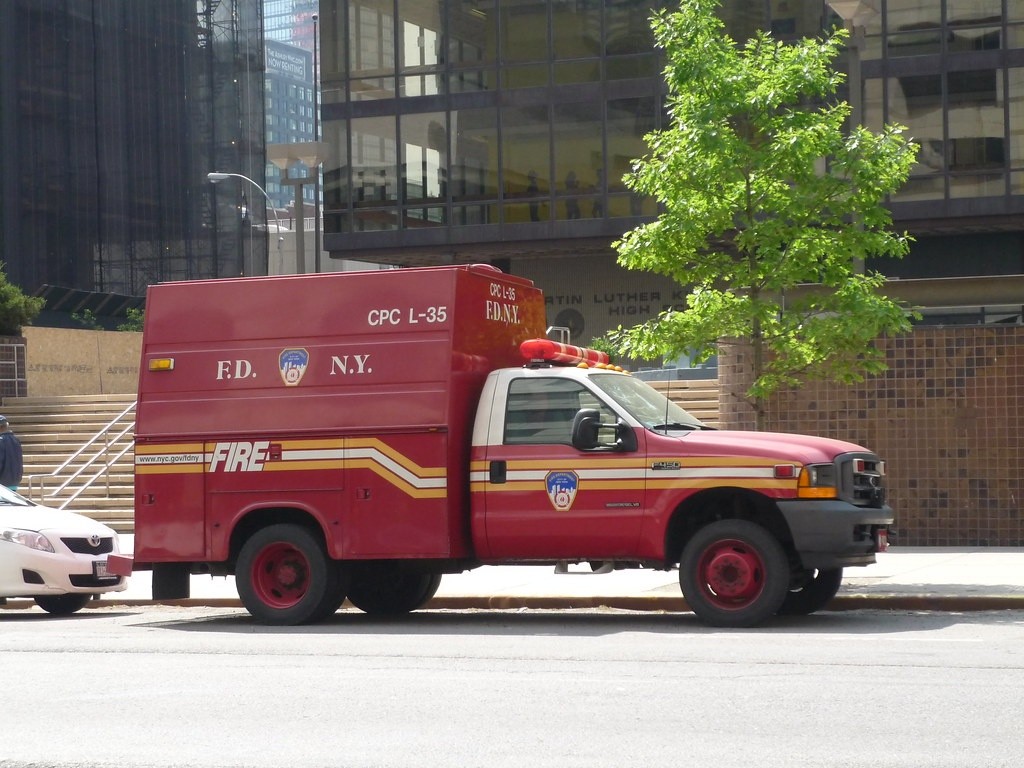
[0,415,8,424]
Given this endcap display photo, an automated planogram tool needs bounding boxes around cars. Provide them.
[1,484,130,615]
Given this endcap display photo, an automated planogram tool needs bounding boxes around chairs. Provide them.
[540,411,571,446]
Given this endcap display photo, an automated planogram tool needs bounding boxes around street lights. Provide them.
[207,171,283,275]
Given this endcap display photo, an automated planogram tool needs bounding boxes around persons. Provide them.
[0,415,22,492]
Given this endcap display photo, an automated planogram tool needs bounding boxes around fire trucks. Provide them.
[102,262,896,630]
